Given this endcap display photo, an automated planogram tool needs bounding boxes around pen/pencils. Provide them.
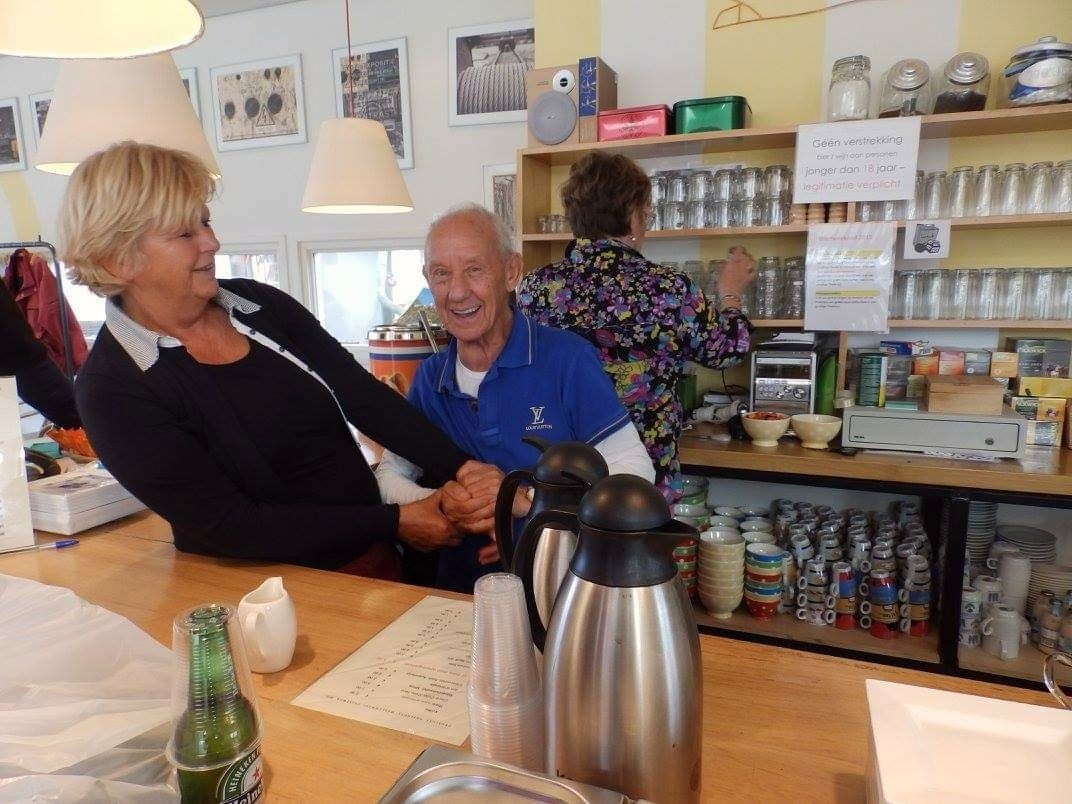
[0,540,79,555]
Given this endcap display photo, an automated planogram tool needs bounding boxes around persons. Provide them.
[375,202,655,575]
[60,140,500,594]
[515,150,759,514]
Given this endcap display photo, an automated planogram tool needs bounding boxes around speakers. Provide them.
[525,64,580,147]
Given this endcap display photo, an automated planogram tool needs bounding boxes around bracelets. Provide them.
[719,295,743,306]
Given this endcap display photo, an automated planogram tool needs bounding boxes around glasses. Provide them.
[642,208,657,231]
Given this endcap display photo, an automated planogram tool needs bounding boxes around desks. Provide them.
[1,508,1072,804]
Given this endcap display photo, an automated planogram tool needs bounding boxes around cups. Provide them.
[660,255,806,319]
[535,163,793,234]
[771,497,933,640]
[889,267,1071,320]
[856,159,1072,223]
[166,604,262,772]
[939,541,1031,660]
[470,572,544,775]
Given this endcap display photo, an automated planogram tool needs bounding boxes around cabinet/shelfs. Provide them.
[515,104,1072,696]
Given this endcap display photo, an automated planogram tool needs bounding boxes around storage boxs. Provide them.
[526,58,672,147]
[878,340,1017,399]
[1006,337,1072,449]
[922,375,1005,416]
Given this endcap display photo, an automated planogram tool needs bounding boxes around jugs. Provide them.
[237,577,298,673]
[493,435,608,658]
[511,475,702,803]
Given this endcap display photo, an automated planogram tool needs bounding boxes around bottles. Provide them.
[827,35,1072,121]
[172,610,265,803]
[1031,589,1072,656]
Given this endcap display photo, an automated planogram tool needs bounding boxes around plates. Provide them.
[940,495,1072,619]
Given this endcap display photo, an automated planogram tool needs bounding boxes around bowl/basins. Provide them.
[742,412,790,447]
[790,414,842,449]
[671,473,783,621]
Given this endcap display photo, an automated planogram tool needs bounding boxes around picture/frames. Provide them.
[330,36,414,170]
[446,17,531,127]
[482,164,518,249]
[179,67,203,130]
[29,89,55,149]
[209,54,306,156]
[0,97,28,173]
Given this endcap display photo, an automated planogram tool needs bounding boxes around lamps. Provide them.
[35,52,222,181]
[0,0,205,60]
[297,0,416,213]
